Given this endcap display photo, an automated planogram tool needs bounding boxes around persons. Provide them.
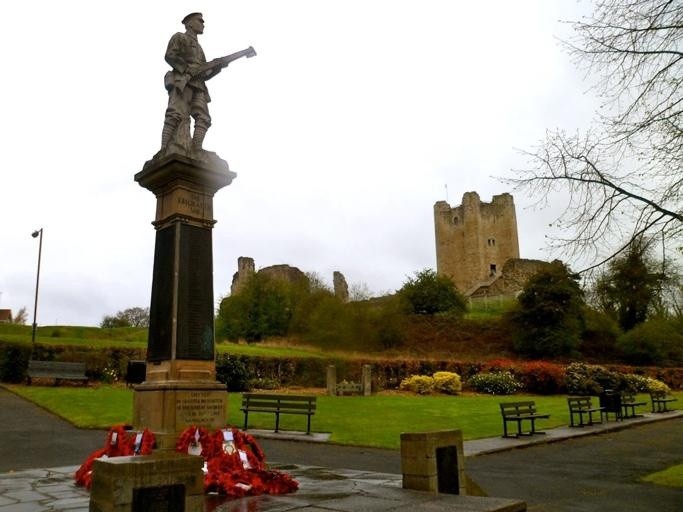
[152,12,228,160]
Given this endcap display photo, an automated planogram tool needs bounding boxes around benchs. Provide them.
[620,393,648,418]
[239,391,317,435]
[26,359,89,387]
[649,390,679,413]
[498,399,552,439]
[567,395,608,427]
